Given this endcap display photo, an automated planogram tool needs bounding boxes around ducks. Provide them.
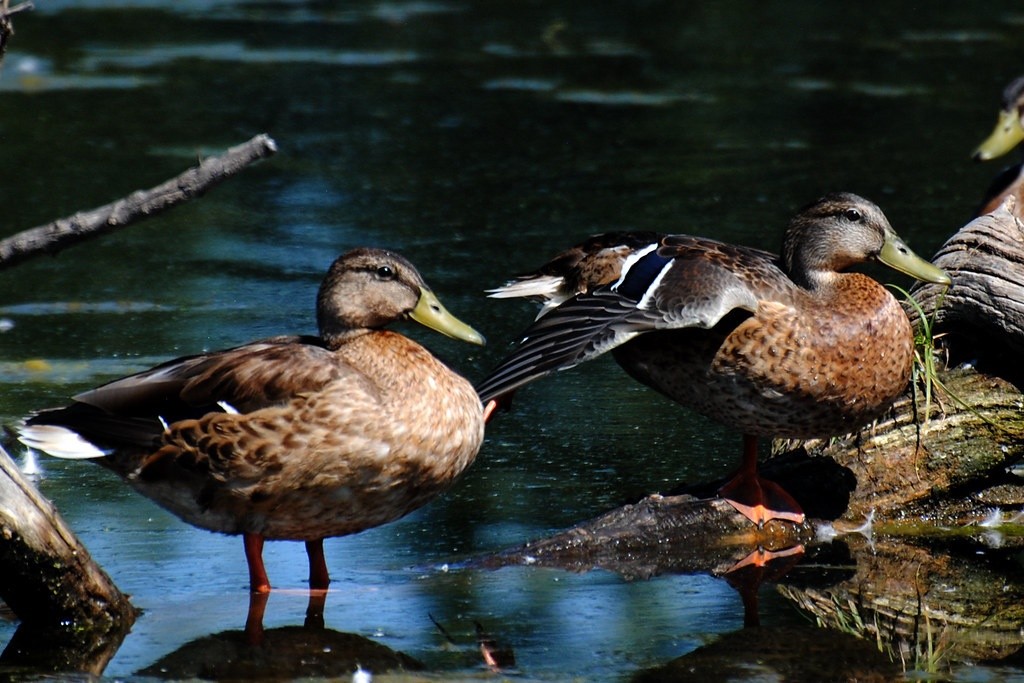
[974,76,1024,223]
[14,249,487,644]
[478,192,951,526]
